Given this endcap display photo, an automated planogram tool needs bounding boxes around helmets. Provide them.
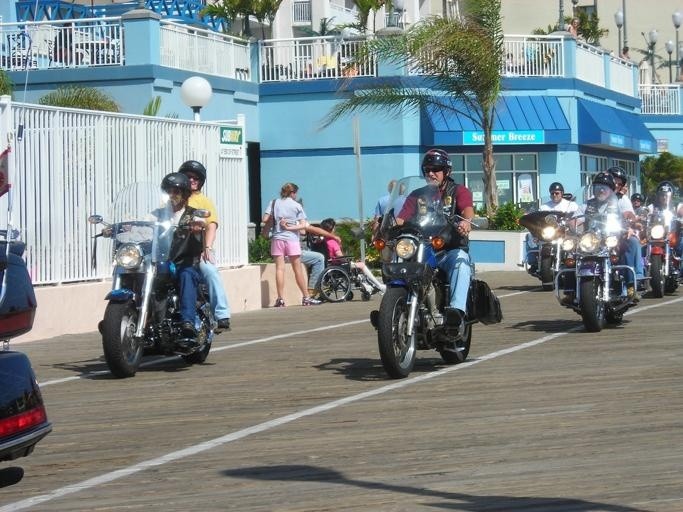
[179,161,206,188]
[608,167,626,187]
[550,182,564,191]
[631,193,642,202]
[161,173,191,199]
[657,182,675,193]
[422,149,452,172]
[593,172,616,190]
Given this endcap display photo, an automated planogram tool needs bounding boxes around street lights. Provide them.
[644,27,662,88]
[664,37,677,87]
[609,11,631,59]
[669,8,683,83]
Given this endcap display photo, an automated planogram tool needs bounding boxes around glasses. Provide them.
[422,167,442,173]
[615,181,624,186]
[551,193,559,195]
[187,174,201,180]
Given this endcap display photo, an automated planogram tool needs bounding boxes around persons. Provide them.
[371,148,475,337]
[260,183,322,307]
[101,172,207,338]
[296,199,341,297]
[179,160,230,329]
[525,166,683,306]
[322,218,387,296]
[372,180,396,282]
[620,46,630,60]
[55,23,84,65]
[568,19,579,38]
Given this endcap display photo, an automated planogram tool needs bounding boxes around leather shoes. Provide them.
[447,309,462,337]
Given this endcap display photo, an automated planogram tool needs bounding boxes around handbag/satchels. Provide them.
[262,200,275,239]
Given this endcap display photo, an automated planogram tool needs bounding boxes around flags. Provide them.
[0,148,9,197]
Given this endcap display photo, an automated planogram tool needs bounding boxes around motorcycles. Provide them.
[1,101,52,483]
[518,201,569,287]
[642,210,682,299]
[364,178,485,376]
[83,176,216,375]
[559,213,638,328]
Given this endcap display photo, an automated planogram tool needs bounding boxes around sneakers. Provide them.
[628,287,642,299]
[274,298,284,306]
[302,297,321,305]
[218,318,229,328]
[183,323,197,337]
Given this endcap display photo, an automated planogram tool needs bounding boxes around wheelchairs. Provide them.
[304,220,372,304]
